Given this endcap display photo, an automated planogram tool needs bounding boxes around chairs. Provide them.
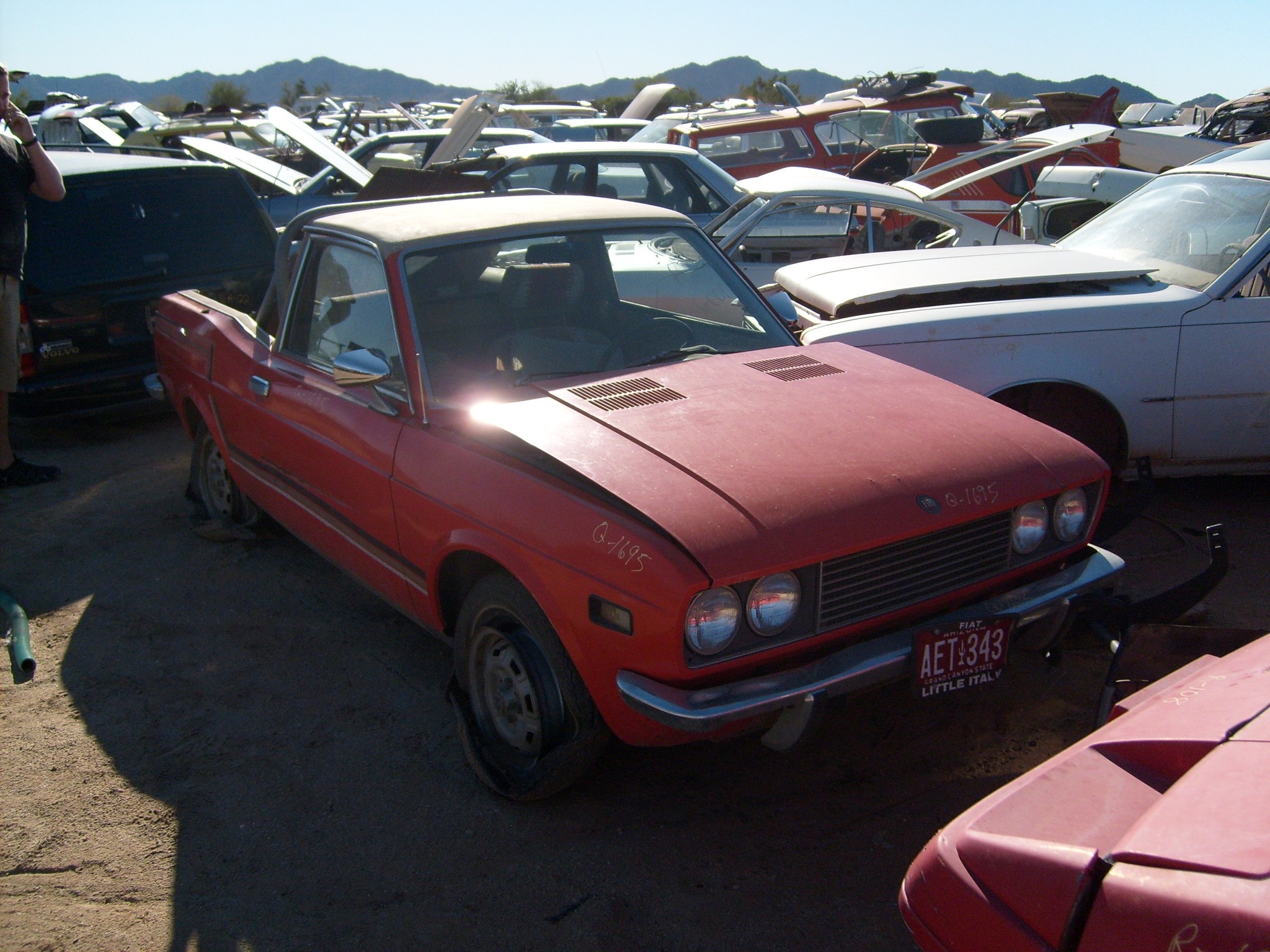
[852,220,884,253]
[416,248,501,362]
[486,263,623,379]
[710,136,801,163]
[567,171,617,198]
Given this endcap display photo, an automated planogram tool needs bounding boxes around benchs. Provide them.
[318,268,505,350]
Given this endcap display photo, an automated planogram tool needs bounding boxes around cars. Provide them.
[743,140,1270,545]
[148,189,1125,805]
[14,69,1268,305]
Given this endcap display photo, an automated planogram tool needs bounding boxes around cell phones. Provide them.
[5,107,19,126]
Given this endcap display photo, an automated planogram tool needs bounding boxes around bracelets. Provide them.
[22,135,38,147]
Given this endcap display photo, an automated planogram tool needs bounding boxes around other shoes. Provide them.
[0,452,62,488]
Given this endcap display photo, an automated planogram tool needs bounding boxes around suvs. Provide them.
[2,144,282,433]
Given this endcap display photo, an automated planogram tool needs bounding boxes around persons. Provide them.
[0,63,67,487]
